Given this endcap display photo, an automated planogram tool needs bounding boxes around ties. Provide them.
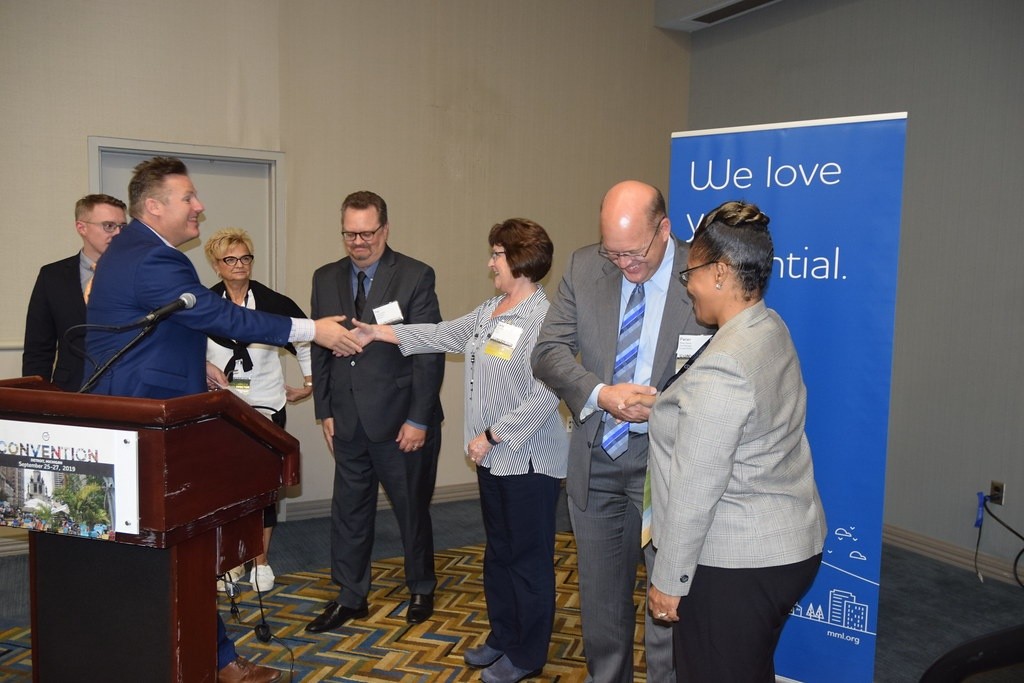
[355,272,366,322]
[602,284,646,460]
[84,263,97,304]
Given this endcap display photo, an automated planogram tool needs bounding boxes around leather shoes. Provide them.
[304,601,369,633]
[219,657,284,683]
[406,595,434,625]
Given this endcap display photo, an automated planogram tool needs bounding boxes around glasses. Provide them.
[342,225,382,240]
[598,216,666,260]
[489,249,505,260]
[83,221,127,233]
[679,261,719,282]
[216,254,254,267]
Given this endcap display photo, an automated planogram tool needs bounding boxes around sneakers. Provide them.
[250,564,276,592]
[217,565,245,592]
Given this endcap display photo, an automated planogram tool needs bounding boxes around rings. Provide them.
[657,610,668,619]
[471,457,475,462]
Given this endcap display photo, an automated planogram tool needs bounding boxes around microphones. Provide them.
[144,293,196,322]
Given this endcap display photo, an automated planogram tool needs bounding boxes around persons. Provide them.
[531,179,717,683]
[203,229,312,592]
[81,157,364,683]
[616,202,826,683]
[306,188,446,633]
[22,194,227,393]
[332,218,570,681]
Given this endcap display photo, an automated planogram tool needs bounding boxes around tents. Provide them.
[22,498,51,512]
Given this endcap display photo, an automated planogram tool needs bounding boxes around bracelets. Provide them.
[303,382,313,387]
[484,427,499,446]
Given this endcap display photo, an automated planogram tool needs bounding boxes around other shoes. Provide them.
[464,644,542,683]
